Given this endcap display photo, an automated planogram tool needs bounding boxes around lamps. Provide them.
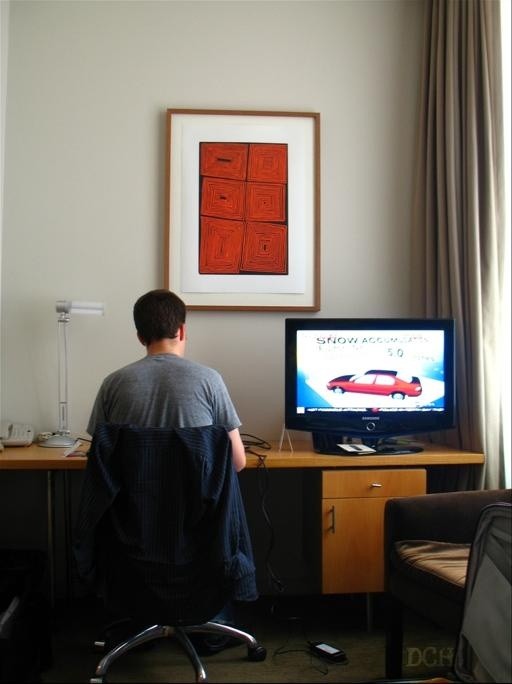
[37,298,109,450]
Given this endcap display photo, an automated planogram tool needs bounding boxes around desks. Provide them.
[1,442,484,642]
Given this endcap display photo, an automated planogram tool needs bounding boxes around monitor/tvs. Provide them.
[285,317,457,455]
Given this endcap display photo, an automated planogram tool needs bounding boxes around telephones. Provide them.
[0,422,34,447]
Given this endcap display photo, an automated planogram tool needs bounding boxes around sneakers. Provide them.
[195,623,254,657]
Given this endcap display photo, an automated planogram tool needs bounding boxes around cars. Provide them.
[324,366,424,403]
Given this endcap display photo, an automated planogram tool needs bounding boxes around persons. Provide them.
[83,287,257,658]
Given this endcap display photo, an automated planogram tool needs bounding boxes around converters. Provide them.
[309,641,346,661]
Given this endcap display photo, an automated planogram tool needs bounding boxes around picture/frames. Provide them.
[161,106,322,313]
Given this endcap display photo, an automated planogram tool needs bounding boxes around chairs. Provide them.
[383,490,511,684]
[86,427,267,683]
